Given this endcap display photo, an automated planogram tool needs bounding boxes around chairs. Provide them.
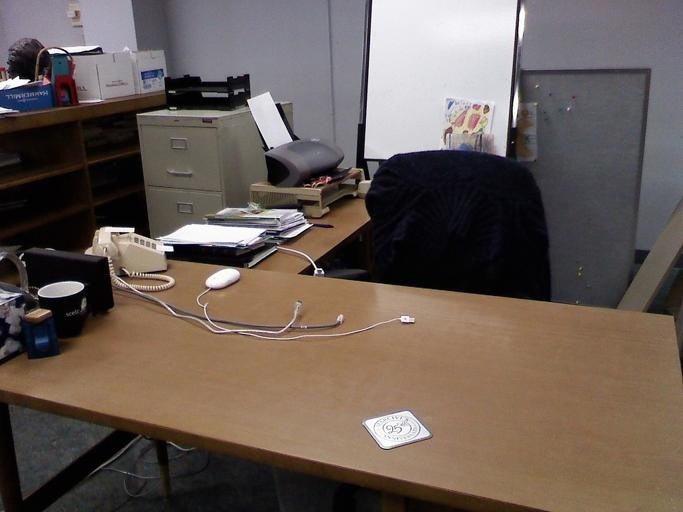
[363,149,554,302]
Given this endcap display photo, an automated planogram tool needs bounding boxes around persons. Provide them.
[5,36,51,82]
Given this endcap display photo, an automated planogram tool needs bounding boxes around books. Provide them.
[151,203,313,269]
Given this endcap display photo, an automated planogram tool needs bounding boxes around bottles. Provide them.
[54,74,80,106]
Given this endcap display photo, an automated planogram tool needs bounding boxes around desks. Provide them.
[0,261,682,511]
[155,196,371,274]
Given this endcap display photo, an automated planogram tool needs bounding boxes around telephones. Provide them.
[85,226,167,277]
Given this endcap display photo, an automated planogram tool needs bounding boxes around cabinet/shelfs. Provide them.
[132,102,293,245]
[0,87,178,252]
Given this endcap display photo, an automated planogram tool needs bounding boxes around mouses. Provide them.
[205,269,240,289]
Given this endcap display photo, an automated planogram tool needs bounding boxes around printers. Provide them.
[264,139,344,187]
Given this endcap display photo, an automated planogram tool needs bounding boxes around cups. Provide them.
[36,280,91,340]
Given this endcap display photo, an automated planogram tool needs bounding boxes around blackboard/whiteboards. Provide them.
[358,0,520,162]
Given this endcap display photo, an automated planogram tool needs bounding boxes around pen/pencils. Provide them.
[313,224,334,228]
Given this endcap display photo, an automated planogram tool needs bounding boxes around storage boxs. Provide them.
[63,50,136,101]
[126,51,170,95]
